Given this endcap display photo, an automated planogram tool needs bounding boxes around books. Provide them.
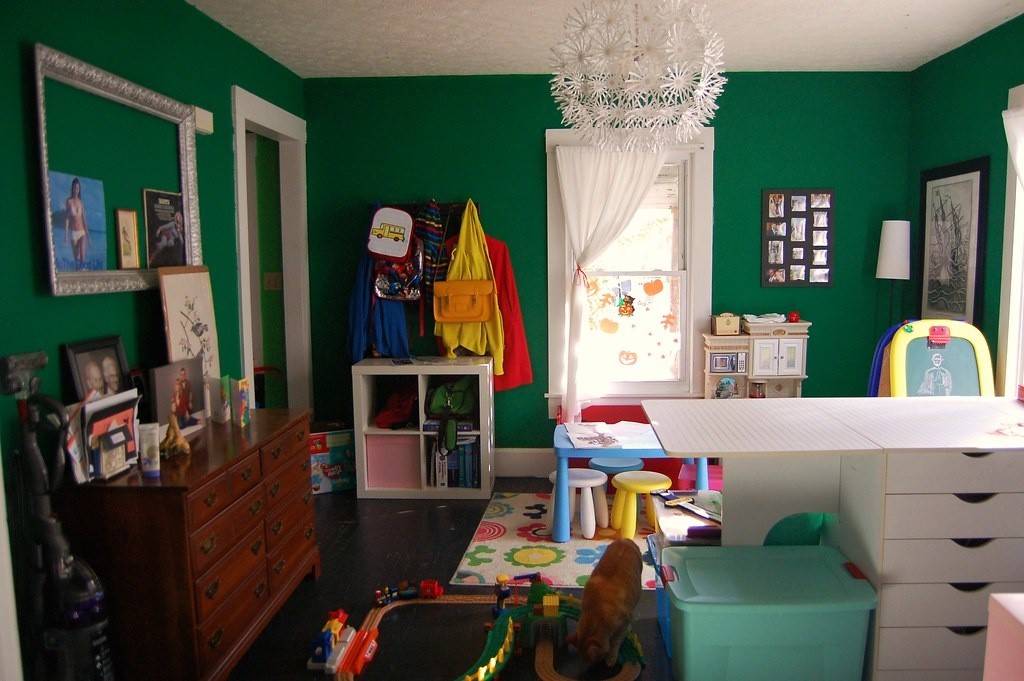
[429,436,481,488]
[424,419,473,431]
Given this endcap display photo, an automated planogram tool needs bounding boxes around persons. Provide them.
[168,368,202,429]
[771,194,782,216]
[64,178,92,271]
[149,212,186,267]
[768,268,782,283]
[84,355,119,400]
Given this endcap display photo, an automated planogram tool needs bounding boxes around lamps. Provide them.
[549,0,728,154]
[875,220,913,328]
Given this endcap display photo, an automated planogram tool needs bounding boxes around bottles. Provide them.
[788,311,799,323]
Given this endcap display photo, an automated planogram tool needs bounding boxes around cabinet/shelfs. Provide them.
[703,320,814,399]
[351,357,497,499]
[642,395,1024,681]
[49,406,319,681]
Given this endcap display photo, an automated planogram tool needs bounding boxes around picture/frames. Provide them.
[62,337,137,406]
[33,43,202,294]
[915,156,988,329]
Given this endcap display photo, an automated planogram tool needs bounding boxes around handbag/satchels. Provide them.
[431,279,498,324]
[375,381,419,429]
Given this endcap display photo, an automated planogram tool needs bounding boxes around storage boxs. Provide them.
[308,430,356,495]
[711,314,740,334]
[643,493,876,681]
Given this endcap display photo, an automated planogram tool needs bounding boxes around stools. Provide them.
[609,470,672,539]
[546,468,609,539]
[589,458,644,514]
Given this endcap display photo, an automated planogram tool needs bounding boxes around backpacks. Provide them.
[424,375,481,456]
[366,206,426,302]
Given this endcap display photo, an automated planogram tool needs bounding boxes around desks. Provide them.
[552,424,708,541]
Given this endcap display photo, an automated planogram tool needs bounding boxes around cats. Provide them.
[565,538,643,667]
